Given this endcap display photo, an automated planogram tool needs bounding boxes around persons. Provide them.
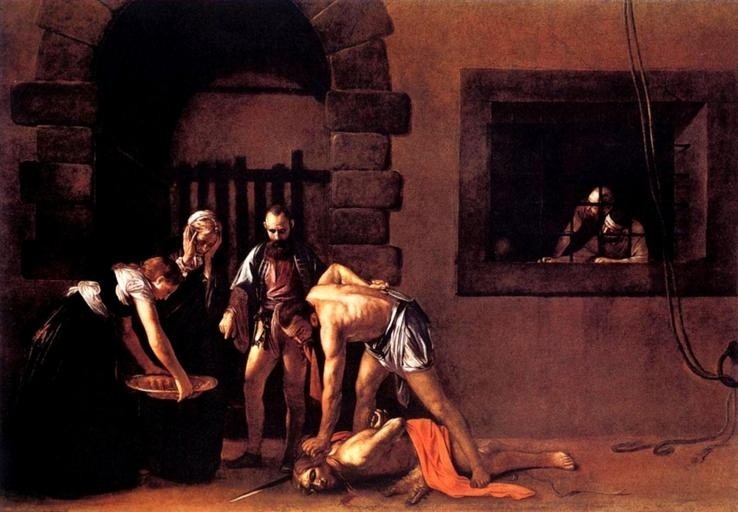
[291,418,577,500]
[15,255,195,501]
[218,203,328,474]
[137,206,229,485]
[552,185,615,258]
[537,207,649,264]
[274,261,493,492]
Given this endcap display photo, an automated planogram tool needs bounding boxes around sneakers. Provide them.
[222,451,262,469]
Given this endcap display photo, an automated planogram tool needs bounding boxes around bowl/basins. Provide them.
[124,372,219,402]
